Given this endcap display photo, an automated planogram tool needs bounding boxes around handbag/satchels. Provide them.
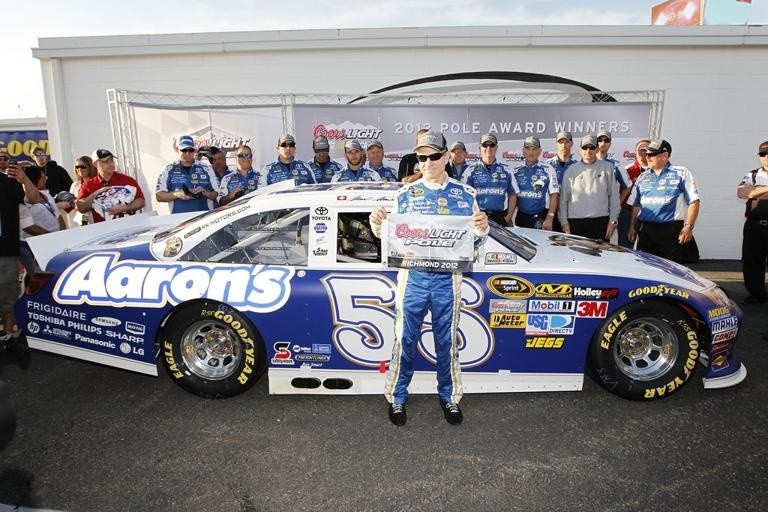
[664,231,700,264]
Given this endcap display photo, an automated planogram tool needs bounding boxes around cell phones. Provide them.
[8,159,18,175]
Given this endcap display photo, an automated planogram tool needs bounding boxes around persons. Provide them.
[1,147,145,370]
[737,141,768,304]
[398,130,699,269]
[369,130,490,423]
[256,133,398,189]
[155,136,259,213]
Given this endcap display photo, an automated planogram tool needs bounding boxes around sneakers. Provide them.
[439,396,464,427]
[742,288,768,306]
[388,401,407,426]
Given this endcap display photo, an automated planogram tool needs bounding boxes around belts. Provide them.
[746,217,768,228]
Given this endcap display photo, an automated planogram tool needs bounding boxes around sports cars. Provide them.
[13,179,748,395]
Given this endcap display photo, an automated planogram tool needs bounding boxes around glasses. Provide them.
[597,138,610,143]
[235,154,253,158]
[582,145,596,151]
[279,142,295,147]
[645,152,665,158]
[481,143,496,148]
[180,148,195,153]
[416,150,446,162]
[758,150,768,157]
[75,165,87,169]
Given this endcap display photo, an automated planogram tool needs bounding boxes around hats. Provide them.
[91,148,119,162]
[597,130,611,141]
[344,139,362,154]
[450,140,466,153]
[556,131,572,143]
[524,136,540,150]
[177,134,195,151]
[0,152,10,159]
[278,134,295,146]
[480,134,498,146]
[313,136,329,150]
[412,130,449,154]
[640,139,672,155]
[367,140,383,151]
[581,133,598,148]
